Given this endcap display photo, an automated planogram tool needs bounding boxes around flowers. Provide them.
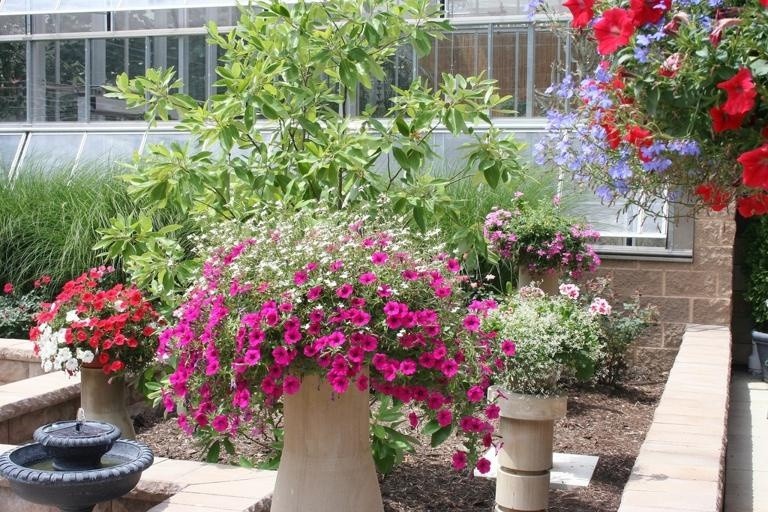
[29,190,628,474]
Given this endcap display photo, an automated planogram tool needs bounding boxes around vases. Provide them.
[270,353,384,512]
[485,384,567,512]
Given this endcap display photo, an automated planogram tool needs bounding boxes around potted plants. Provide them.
[747,210,768,380]
